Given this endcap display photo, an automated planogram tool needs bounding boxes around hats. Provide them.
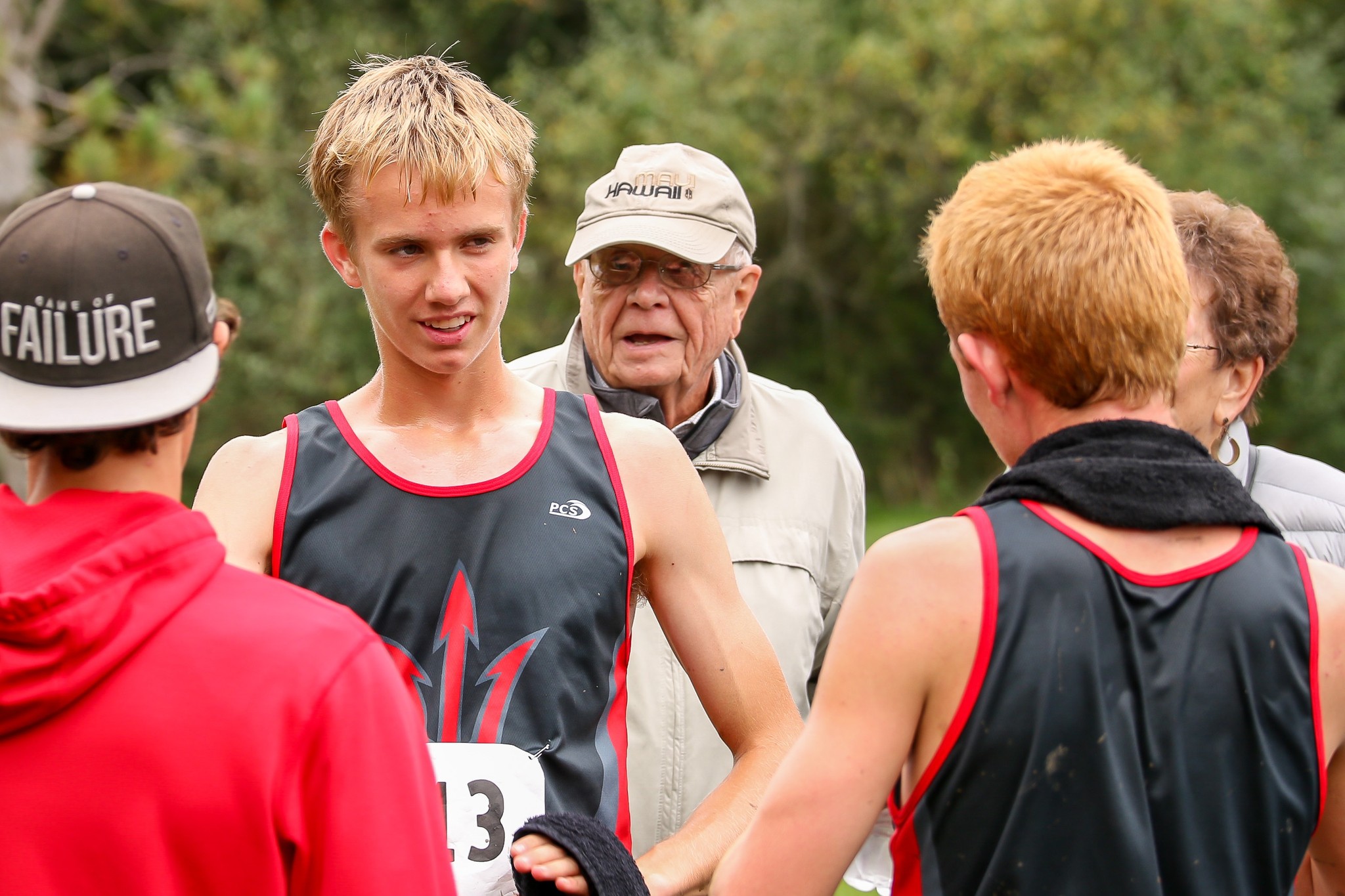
[0,180,220,432]
[564,142,756,267]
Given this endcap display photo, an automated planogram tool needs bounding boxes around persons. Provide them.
[506,142,865,860]
[188,55,804,895]
[710,141,1344,896]
[1,181,459,896]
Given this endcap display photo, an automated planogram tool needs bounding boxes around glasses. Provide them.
[584,246,744,289]
